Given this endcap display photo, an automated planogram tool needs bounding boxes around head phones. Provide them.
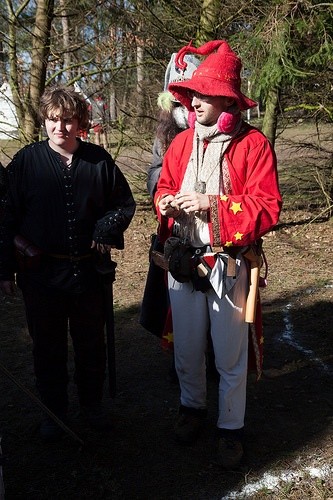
[188,110,242,134]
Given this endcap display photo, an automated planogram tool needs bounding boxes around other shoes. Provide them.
[180,409,208,448]
[206,356,220,382]
[215,436,244,469]
[166,356,180,387]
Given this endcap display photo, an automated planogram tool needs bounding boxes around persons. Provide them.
[77,94,109,149]
[140,54,206,387]
[0,86,136,442]
[152,41,282,455]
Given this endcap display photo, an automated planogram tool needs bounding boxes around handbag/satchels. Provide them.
[139,235,169,337]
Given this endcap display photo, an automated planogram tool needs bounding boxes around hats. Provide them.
[155,53,201,112]
[167,40,258,112]
